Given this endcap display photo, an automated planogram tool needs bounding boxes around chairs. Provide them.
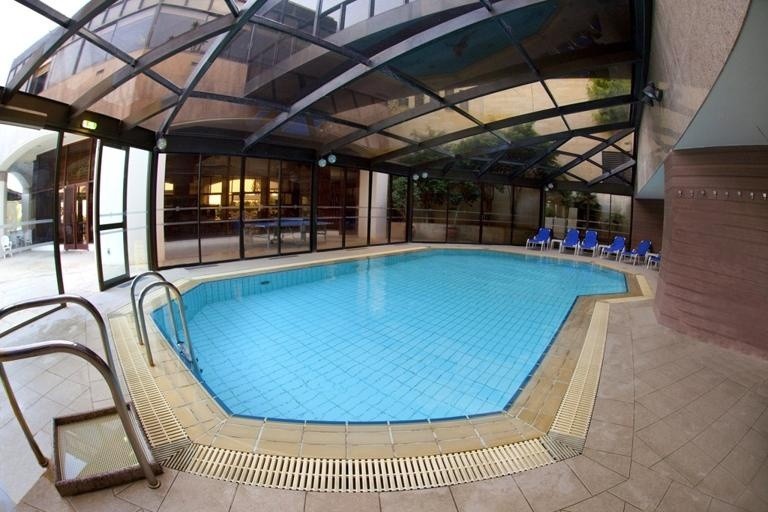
[526,227,661,271]
[1,230,31,259]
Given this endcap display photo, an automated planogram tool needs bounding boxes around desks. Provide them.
[243,221,334,250]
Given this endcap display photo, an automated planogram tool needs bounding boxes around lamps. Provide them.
[318,151,337,168]
[640,81,663,107]
[543,182,557,192]
[412,171,430,183]
[154,131,168,152]
